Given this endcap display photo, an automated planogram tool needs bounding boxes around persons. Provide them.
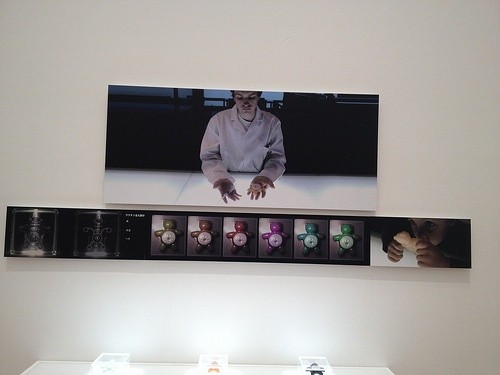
[199,90,288,204]
[382,216,470,268]
